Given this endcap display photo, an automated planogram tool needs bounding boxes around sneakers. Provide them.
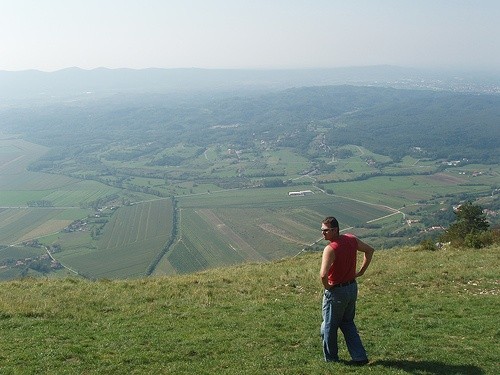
[349,360,370,368]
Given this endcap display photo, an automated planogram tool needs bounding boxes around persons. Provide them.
[320,216,375,366]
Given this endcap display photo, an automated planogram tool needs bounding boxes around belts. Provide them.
[329,278,356,289]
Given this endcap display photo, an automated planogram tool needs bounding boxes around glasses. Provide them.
[320,227,335,233]
